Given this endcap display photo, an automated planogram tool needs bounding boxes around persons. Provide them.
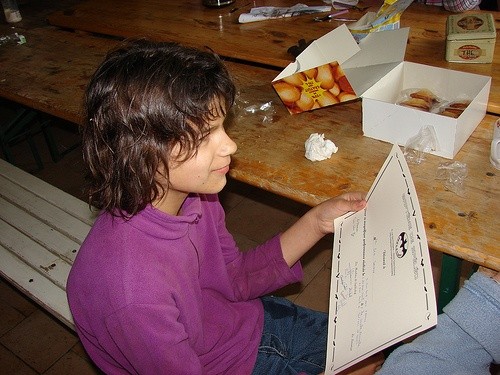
[66,37,383,375]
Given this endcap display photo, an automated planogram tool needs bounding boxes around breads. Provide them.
[401,90,471,119]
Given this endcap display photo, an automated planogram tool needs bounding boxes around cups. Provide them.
[489,119,500,171]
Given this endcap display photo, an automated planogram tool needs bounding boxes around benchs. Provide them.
[0,158,99,333]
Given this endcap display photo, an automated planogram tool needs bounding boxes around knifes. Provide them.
[312,10,348,22]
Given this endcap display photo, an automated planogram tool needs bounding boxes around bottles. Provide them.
[1,0,22,23]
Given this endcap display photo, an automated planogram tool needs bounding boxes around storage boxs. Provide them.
[360,61,492,160]
[446,13,496,63]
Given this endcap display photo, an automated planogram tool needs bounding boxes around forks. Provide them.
[334,2,368,11]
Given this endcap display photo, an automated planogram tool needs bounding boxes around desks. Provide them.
[0,0,500,317]
